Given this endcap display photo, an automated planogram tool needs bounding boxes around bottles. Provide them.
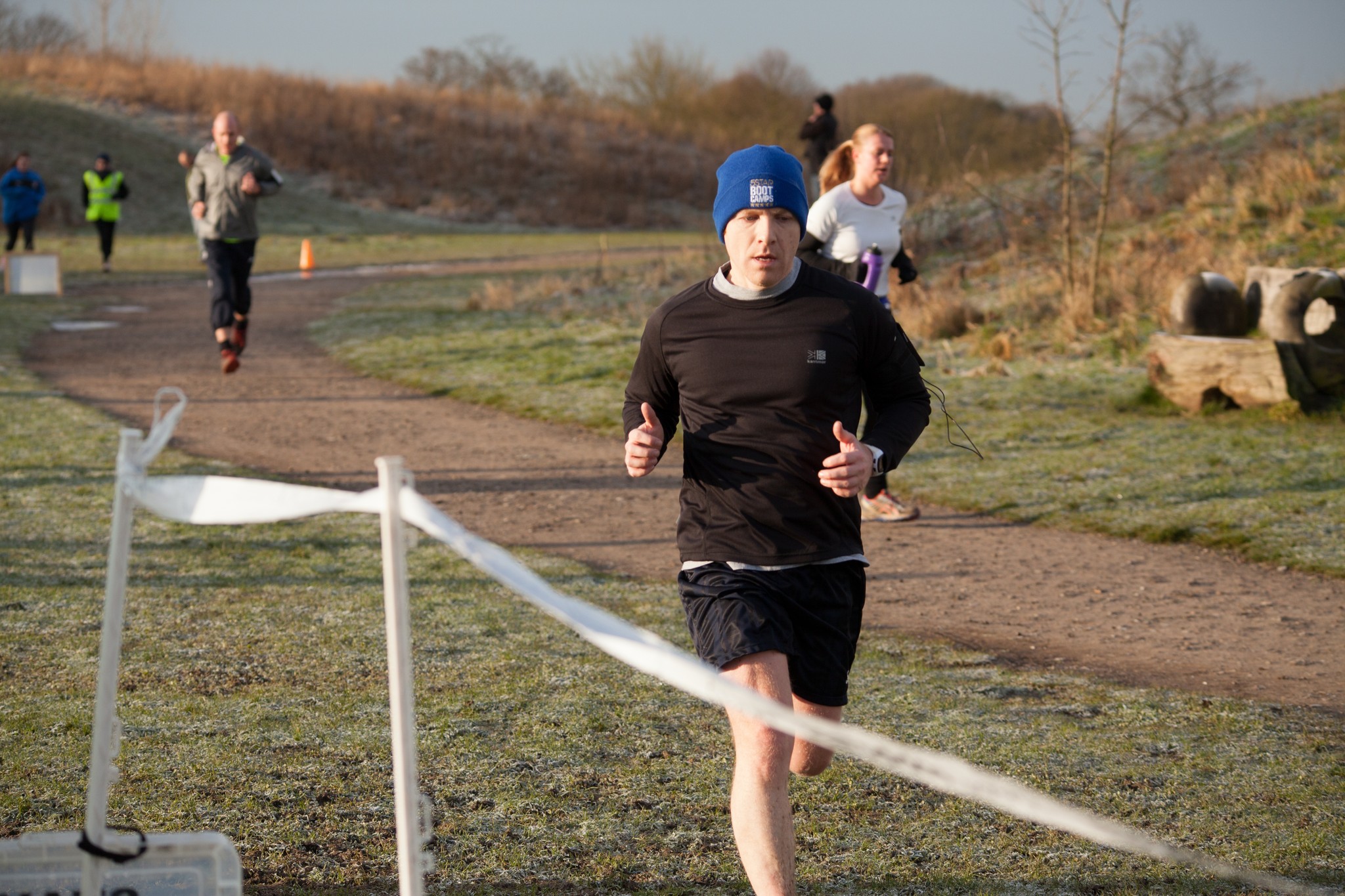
[853,244,884,293]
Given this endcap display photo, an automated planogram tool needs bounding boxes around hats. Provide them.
[713,146,809,247]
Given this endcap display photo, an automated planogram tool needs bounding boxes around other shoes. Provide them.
[103,261,111,273]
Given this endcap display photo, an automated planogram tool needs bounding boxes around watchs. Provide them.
[863,443,884,477]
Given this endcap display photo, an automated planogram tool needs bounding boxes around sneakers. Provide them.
[860,488,919,522]
[222,350,240,373]
[230,320,249,354]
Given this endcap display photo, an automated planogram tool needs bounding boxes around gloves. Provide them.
[891,252,918,284]
[844,247,880,281]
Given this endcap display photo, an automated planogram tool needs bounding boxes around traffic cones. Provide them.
[297,237,316,270]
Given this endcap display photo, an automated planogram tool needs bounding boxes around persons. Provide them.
[799,94,924,521]
[188,110,285,374]
[623,145,933,896]
[0,149,214,286]
